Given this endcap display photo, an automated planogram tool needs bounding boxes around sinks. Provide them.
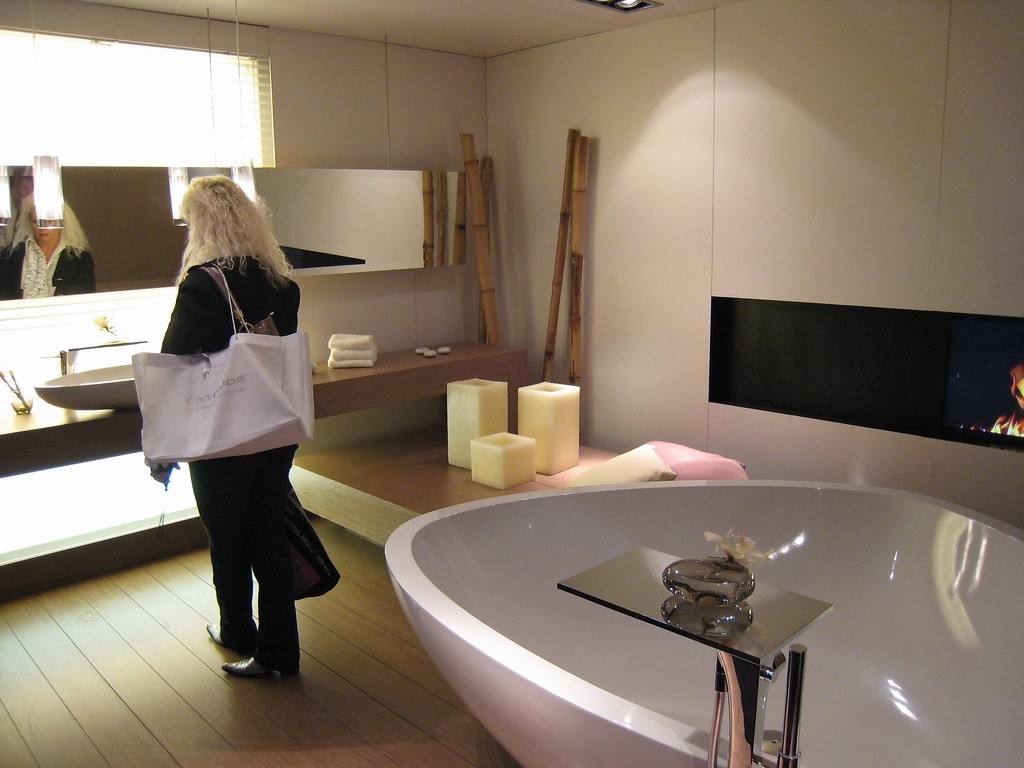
[35,364,140,410]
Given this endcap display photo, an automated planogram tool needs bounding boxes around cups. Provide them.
[7,388,34,415]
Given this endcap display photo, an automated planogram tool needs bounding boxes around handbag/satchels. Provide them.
[131,262,314,455]
[203,263,282,339]
[284,475,341,600]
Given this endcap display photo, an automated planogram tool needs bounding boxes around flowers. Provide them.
[704,528,769,565]
[92,314,118,337]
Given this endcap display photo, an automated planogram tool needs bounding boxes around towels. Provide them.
[328,334,377,367]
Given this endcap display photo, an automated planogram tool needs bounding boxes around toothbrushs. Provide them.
[0,370,29,408]
[8,370,23,396]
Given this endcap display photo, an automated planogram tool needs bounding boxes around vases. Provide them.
[662,558,755,606]
[660,596,754,640]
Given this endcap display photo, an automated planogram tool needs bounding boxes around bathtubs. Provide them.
[385,480,1024,767]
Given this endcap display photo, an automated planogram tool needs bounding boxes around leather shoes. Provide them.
[221,655,298,677]
[207,622,223,645]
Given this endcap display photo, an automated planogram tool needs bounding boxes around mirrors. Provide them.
[0,165,425,301]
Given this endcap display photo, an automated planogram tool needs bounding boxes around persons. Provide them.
[0,193,96,300]
[144,175,341,677]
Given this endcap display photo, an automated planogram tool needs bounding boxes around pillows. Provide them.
[567,444,677,488]
[647,440,748,481]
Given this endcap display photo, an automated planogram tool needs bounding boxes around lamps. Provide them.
[230,158,257,200]
[32,154,64,228]
[0,165,13,226]
[168,167,189,227]
